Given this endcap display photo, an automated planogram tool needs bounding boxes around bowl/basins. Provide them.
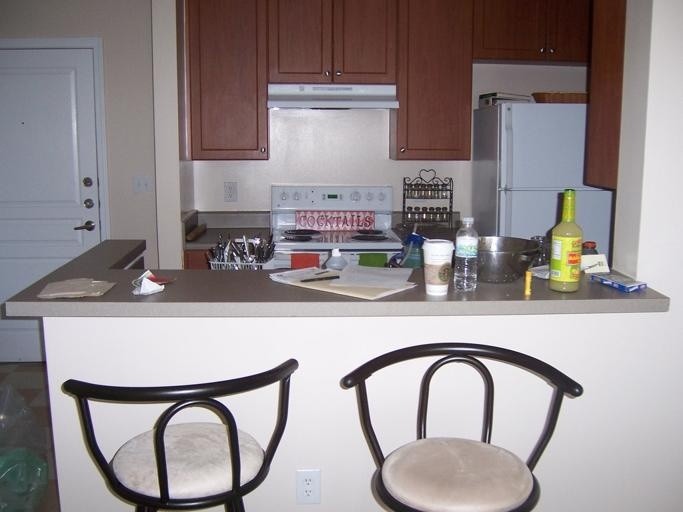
[477,236,541,284]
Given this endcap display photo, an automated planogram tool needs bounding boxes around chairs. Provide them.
[60,357,299,512]
[341,342,584,512]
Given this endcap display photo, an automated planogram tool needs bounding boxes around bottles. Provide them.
[454,216,480,294]
[549,189,583,292]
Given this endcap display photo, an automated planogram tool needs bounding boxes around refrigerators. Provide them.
[474,101,613,266]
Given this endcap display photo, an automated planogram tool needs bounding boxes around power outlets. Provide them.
[296,469,322,505]
[224,181,237,203]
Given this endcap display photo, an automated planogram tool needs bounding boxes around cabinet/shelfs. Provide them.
[267,0,397,84]
[473,0,592,65]
[186,0,269,161]
[389,0,473,161]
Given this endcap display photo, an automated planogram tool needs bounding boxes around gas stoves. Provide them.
[270,185,405,263]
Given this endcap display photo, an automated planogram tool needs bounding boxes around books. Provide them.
[269,266,417,300]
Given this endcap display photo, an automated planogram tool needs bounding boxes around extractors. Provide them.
[266,83,400,111]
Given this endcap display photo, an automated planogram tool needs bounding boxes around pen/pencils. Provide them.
[300,275,340,282]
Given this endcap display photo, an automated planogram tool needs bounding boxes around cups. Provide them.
[420,239,455,297]
